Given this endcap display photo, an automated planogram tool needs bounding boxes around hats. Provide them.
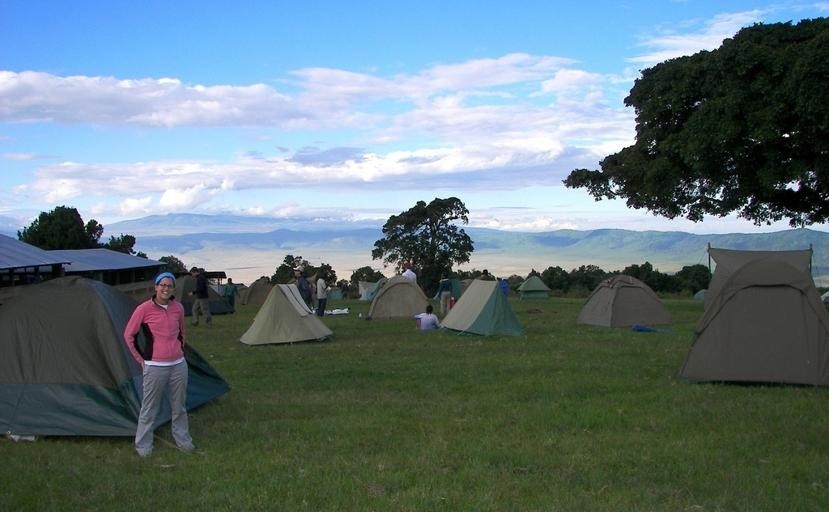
[293,266,304,272]
[155,272,176,287]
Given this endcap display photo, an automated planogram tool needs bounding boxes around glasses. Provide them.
[159,282,173,288]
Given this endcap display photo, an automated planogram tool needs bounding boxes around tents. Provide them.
[244,278,272,305]
[679,243,829,389]
[576,274,673,329]
[517,275,552,298]
[694,289,707,300]
[175,275,236,315]
[1,276,231,441]
[239,284,333,346]
[328,286,343,300]
[821,291,828,302]
[433,279,472,303]
[235,286,249,304]
[441,279,524,336]
[368,277,430,320]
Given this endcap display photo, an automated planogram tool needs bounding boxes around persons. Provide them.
[223,278,240,314]
[402,262,417,284]
[292,265,312,305]
[316,274,329,316]
[438,272,452,314]
[188,265,212,326]
[341,283,349,299]
[500,279,509,299]
[124,272,194,454]
[479,269,495,280]
[413,305,440,329]
